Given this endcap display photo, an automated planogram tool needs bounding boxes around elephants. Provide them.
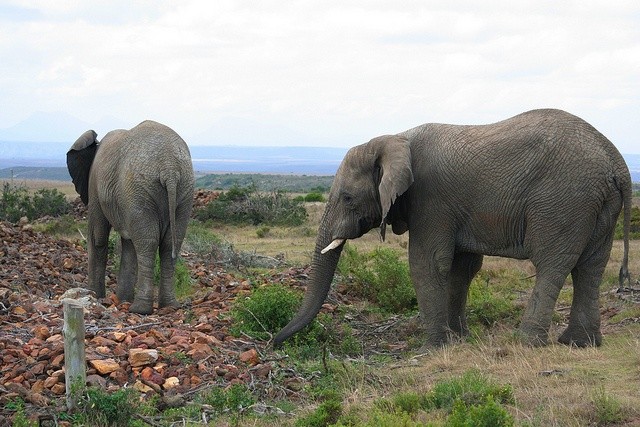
[65,120,196,316]
[270,107,633,356]
